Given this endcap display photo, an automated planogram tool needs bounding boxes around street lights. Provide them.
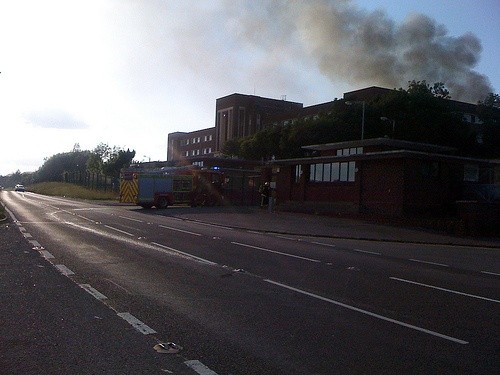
[345,100,396,141]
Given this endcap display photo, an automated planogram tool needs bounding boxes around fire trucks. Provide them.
[120,165,220,208]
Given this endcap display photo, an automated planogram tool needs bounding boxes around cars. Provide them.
[15,184,24,192]
